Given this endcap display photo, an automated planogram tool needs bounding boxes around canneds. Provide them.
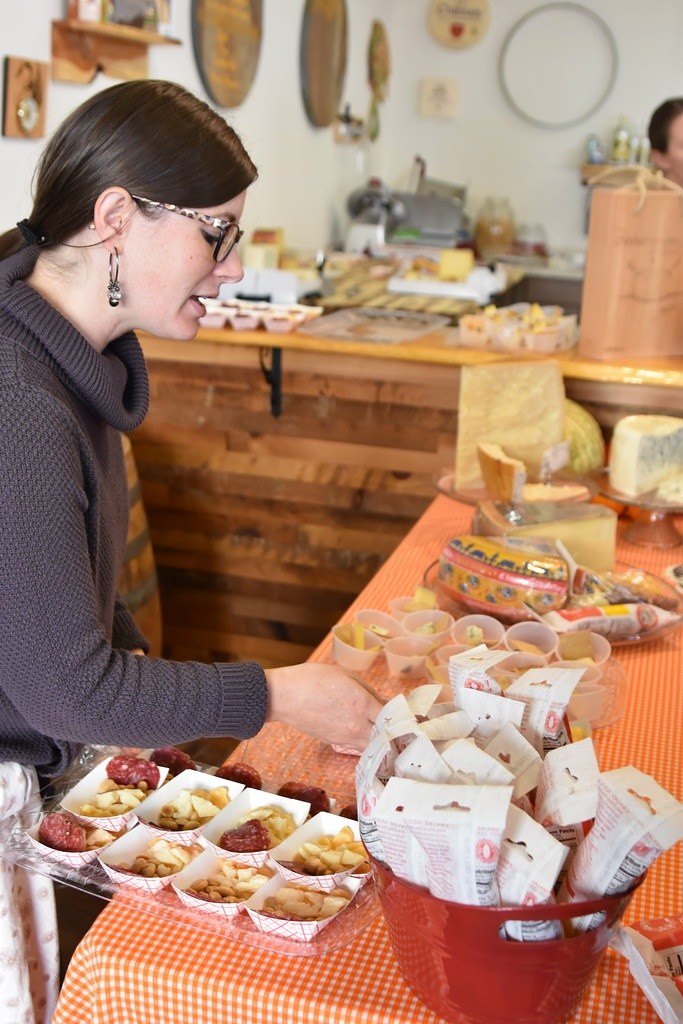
[608,125,651,166]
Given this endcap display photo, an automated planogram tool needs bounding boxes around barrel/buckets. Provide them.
[360,833,646,1023]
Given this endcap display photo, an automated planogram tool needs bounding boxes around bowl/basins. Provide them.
[457,303,577,353]
[331,595,611,721]
[198,299,323,334]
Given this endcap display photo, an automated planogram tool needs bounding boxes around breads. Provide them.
[608,414,683,495]
[437,536,567,615]
[475,443,588,503]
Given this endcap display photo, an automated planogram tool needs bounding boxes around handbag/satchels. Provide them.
[580,163,683,362]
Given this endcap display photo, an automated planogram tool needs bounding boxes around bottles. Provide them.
[513,222,548,265]
[353,178,383,216]
[476,197,514,260]
[587,132,652,165]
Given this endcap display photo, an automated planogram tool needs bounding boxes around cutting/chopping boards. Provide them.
[191,0,263,109]
[300,1,347,128]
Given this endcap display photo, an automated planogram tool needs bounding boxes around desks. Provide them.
[50,449,683,1024]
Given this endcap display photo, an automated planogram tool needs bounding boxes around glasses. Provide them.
[89,194,244,263]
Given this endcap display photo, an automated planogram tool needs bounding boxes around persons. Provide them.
[648,96,683,187]
[0,82,384,1024]
